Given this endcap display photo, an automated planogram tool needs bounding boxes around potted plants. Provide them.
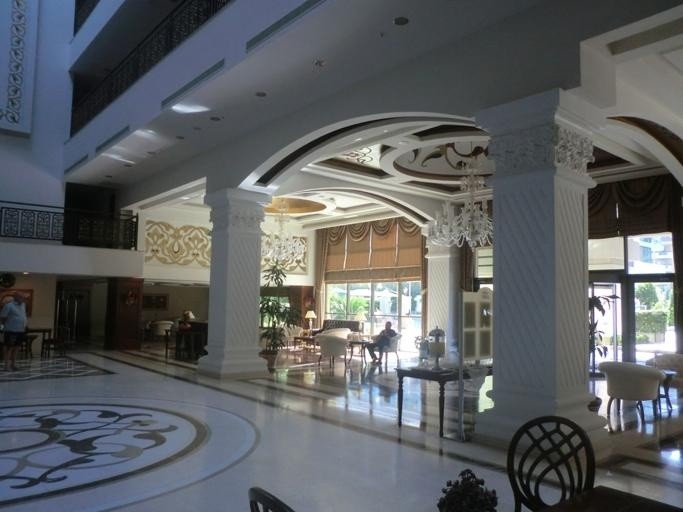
[587,279,621,412]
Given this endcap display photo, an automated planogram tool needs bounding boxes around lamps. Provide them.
[305,310,317,330]
[259,199,304,262]
[428,326,446,373]
[423,160,494,248]
[259,258,301,370]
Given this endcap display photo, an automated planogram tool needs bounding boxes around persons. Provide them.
[0,289,28,371]
[178,310,196,331]
[367,321,397,363]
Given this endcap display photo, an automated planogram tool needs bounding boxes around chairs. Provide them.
[596,351,683,432]
[148,321,174,344]
[17,335,37,359]
[506,414,595,512]
[315,327,401,365]
[43,327,67,358]
[164,329,203,362]
[247,486,294,512]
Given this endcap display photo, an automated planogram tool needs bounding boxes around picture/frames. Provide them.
[0,288,33,318]
[142,293,169,310]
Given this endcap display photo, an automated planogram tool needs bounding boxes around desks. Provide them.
[544,485,683,512]
[393,363,492,438]
[26,328,51,360]
[178,320,208,356]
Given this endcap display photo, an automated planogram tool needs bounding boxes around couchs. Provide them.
[303,319,364,344]
